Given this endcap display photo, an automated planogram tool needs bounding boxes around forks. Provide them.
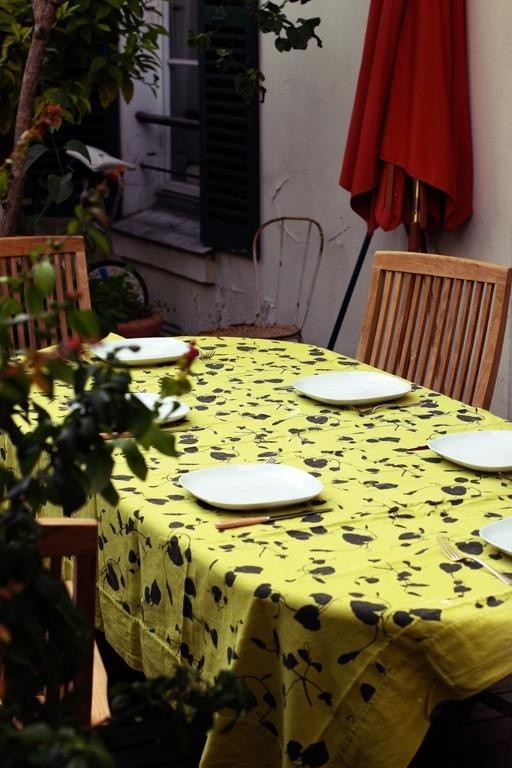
[265,457,280,464]
[438,537,511,588]
[197,347,215,360]
[347,400,427,415]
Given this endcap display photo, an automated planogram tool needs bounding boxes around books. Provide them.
[65,141,138,173]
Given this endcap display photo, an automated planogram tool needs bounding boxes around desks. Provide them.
[5,336,512,768]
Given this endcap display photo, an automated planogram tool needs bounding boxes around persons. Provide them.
[12,89,129,230]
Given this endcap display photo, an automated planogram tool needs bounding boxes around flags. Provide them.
[336,0,479,256]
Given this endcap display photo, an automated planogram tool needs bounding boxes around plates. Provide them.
[479,517,512,557]
[293,369,411,408]
[427,429,511,473]
[178,462,324,510]
[68,392,187,430]
[92,337,191,368]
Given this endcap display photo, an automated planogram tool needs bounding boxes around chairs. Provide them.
[197,216,323,340]
[1,236,100,356]
[355,251,511,413]
[1,515,117,742]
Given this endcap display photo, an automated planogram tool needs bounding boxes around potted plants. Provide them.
[94,263,173,337]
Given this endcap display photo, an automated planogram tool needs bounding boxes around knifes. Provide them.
[100,427,198,440]
[214,507,334,530]
